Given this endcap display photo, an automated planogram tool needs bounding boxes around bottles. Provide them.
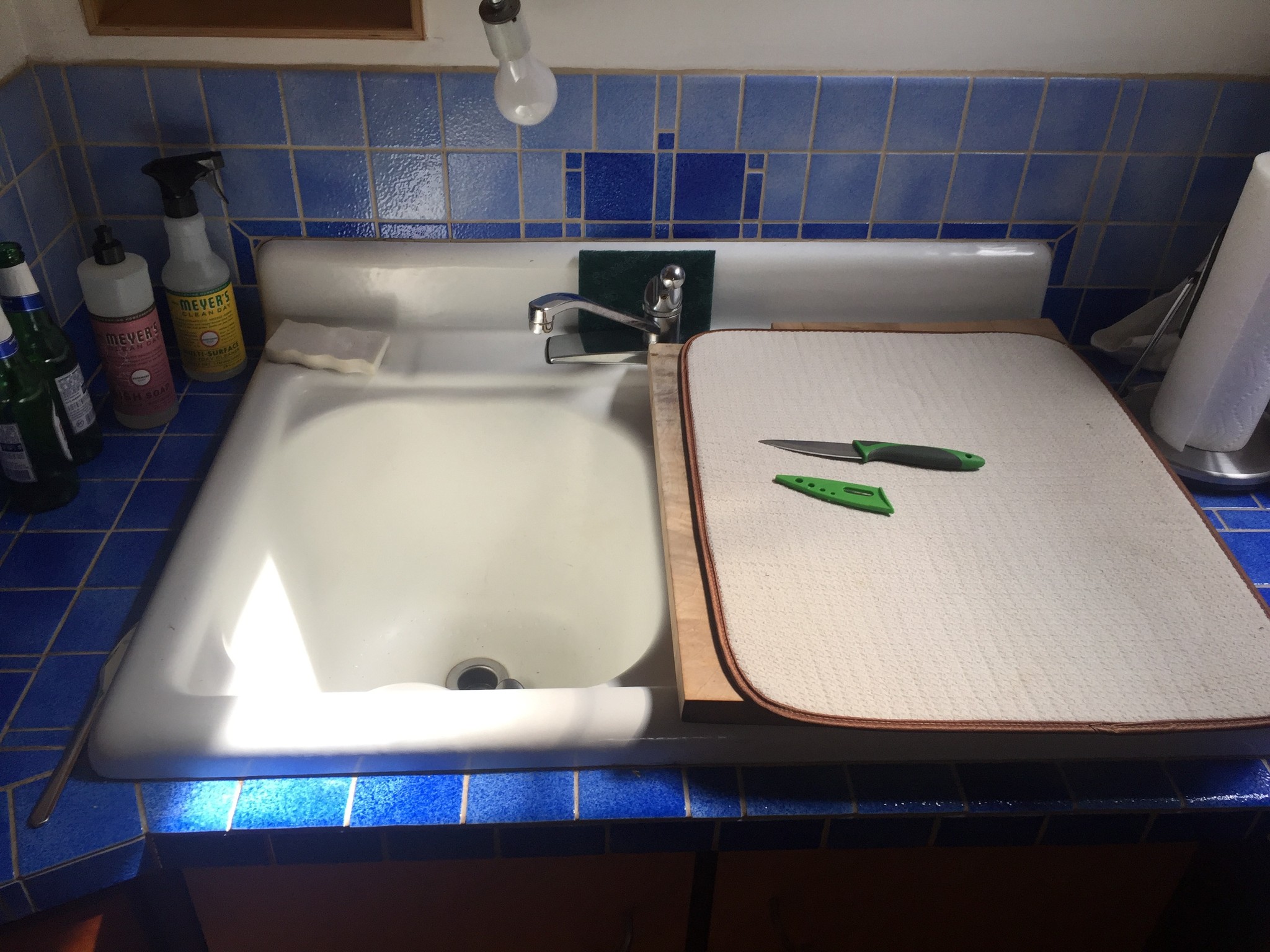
[0,240,106,461]
[0,302,80,512]
[77,223,179,429]
[148,148,246,380]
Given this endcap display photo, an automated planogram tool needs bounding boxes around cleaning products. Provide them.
[141,151,256,384]
[73,221,184,434]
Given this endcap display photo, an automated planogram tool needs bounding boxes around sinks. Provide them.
[85,231,1270,776]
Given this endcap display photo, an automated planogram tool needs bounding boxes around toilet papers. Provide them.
[1145,145,1270,461]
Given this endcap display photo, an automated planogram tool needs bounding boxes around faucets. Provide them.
[527,259,688,373]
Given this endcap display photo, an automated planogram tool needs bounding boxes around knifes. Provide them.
[758,440,984,472]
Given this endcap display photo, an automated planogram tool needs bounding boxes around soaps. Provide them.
[261,317,394,376]
